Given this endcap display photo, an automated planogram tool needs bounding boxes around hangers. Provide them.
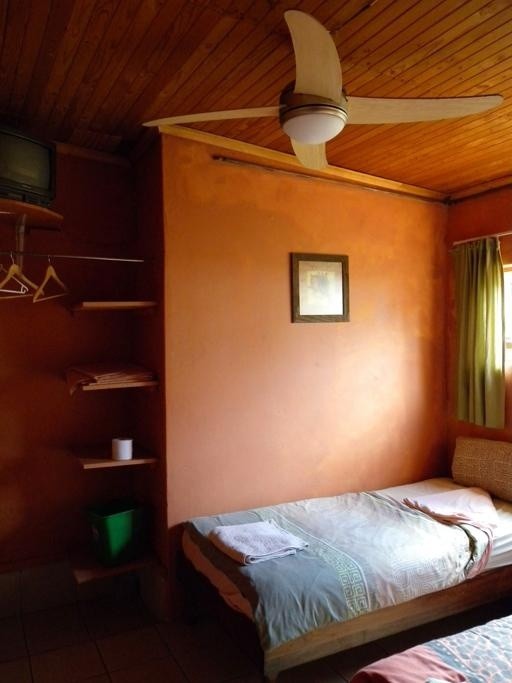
[0,249,72,304]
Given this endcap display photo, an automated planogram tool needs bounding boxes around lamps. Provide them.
[278,104,349,144]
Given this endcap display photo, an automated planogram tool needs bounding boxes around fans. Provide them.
[139,7,501,170]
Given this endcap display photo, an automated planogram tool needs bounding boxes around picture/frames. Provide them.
[289,252,350,324]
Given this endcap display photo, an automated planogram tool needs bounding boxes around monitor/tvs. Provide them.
[0,125,57,207]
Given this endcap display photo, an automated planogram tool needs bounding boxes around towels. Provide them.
[208,518,310,565]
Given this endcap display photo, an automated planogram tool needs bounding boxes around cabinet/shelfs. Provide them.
[65,298,159,584]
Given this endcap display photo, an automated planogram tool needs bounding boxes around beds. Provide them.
[178,418,512,682]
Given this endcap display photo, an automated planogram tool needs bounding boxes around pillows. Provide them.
[451,434,512,504]
